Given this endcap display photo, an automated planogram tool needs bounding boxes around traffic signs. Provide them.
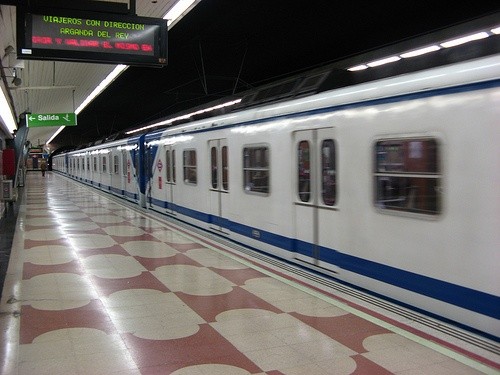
[26,113,77,128]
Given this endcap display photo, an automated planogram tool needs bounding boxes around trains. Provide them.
[52,54,500,341]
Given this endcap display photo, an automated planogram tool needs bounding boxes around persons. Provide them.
[41,162,46,177]
